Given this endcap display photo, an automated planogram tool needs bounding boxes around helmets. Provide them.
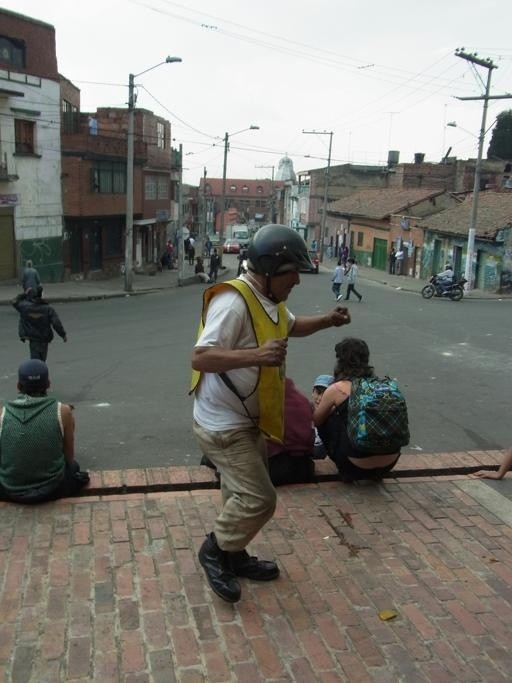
[444,264,453,270]
[246,221,317,276]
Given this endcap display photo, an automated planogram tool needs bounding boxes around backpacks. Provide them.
[338,374,411,450]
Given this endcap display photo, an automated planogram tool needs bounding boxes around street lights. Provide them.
[216,124,265,272]
[446,121,482,293]
[115,50,195,298]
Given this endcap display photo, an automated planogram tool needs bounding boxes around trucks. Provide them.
[224,224,251,249]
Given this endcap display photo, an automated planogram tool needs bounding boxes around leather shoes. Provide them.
[197,530,243,605]
[230,548,282,581]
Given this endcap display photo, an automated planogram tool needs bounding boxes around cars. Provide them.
[220,239,242,254]
[298,248,322,277]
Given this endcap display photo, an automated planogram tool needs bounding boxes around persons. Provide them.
[187,224,351,603]
[166,229,249,284]
[434,264,453,297]
[473,443,512,480]
[311,239,362,302]
[388,248,397,275]
[395,247,404,276]
[199,337,401,484]
[21,259,41,294]
[9,286,67,364]
[0,359,80,505]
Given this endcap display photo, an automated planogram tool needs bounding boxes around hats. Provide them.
[312,374,337,389]
[16,358,50,383]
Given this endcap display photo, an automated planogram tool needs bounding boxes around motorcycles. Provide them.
[421,271,466,303]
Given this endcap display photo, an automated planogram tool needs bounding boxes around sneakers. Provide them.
[441,290,449,295]
[334,294,363,302]
